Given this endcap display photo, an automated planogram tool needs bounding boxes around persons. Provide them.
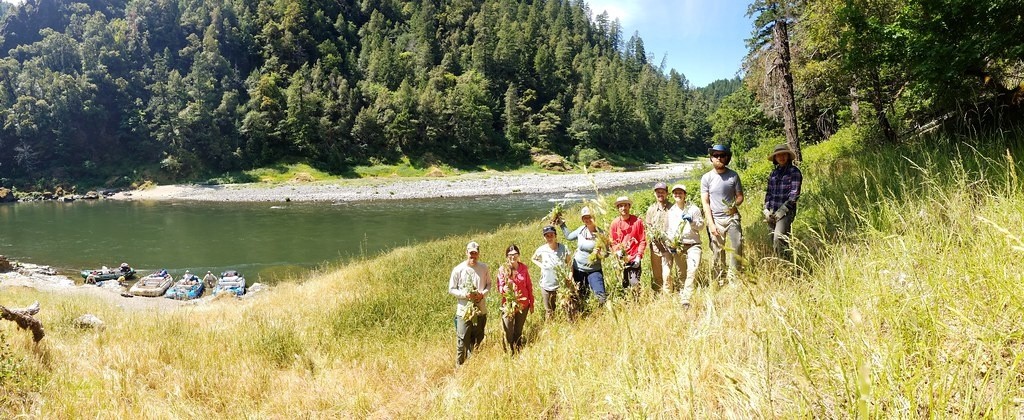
[235,273,247,295]
[160,268,168,278]
[764,144,803,270]
[15,260,19,267]
[119,263,129,272]
[183,270,200,282]
[556,205,610,307]
[666,183,704,312]
[202,271,217,288]
[644,182,674,301]
[86,275,96,285]
[116,274,125,285]
[530,225,572,324]
[449,242,492,366]
[497,244,535,356]
[701,144,744,294]
[608,196,647,302]
[92,270,98,275]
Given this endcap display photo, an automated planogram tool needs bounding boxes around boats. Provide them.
[130,268,174,298]
[164,270,204,300]
[212,269,246,297]
[80,262,136,283]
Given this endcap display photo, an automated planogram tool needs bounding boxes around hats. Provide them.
[654,183,668,192]
[467,242,479,253]
[581,206,590,216]
[672,185,687,193]
[767,145,796,161]
[614,197,632,207]
[543,226,556,234]
[707,144,728,155]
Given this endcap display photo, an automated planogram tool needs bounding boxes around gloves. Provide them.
[774,204,789,222]
[681,214,692,222]
[667,246,677,255]
[763,208,775,224]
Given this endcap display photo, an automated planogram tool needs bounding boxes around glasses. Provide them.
[710,153,726,159]
[508,253,519,256]
